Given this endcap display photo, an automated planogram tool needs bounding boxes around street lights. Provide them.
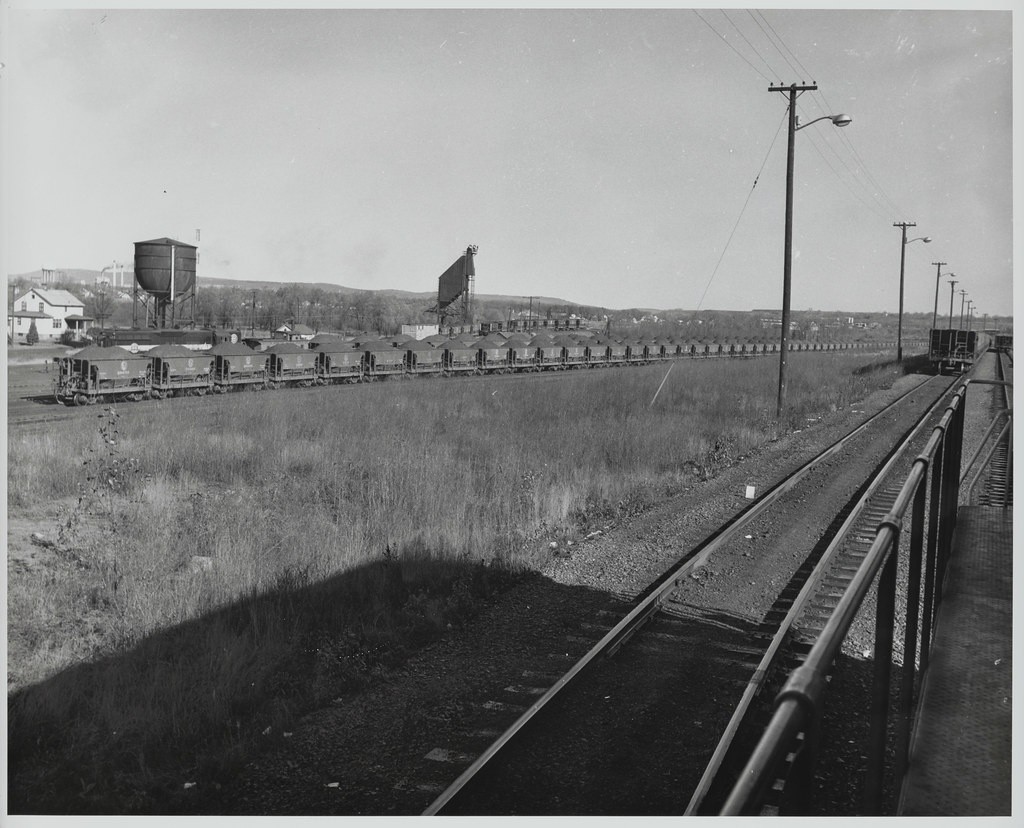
[898,237,933,362]
[950,289,980,330]
[776,113,853,416]
[934,273,956,328]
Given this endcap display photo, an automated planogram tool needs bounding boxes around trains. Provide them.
[993,334,1013,353]
[930,328,991,373]
[52,330,929,407]
